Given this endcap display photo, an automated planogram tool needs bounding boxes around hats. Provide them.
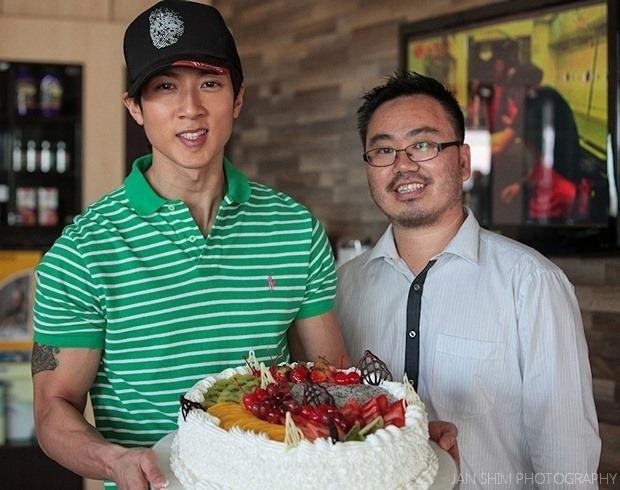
[123,0,240,96]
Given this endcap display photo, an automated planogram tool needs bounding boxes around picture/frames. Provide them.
[399,1,618,259]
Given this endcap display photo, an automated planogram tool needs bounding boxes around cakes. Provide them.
[167,343,440,490]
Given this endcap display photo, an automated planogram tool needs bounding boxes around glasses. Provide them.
[363,141,460,167]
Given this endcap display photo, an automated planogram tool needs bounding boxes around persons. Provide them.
[300,68,603,490]
[31,0,460,489]
[492,63,582,226]
